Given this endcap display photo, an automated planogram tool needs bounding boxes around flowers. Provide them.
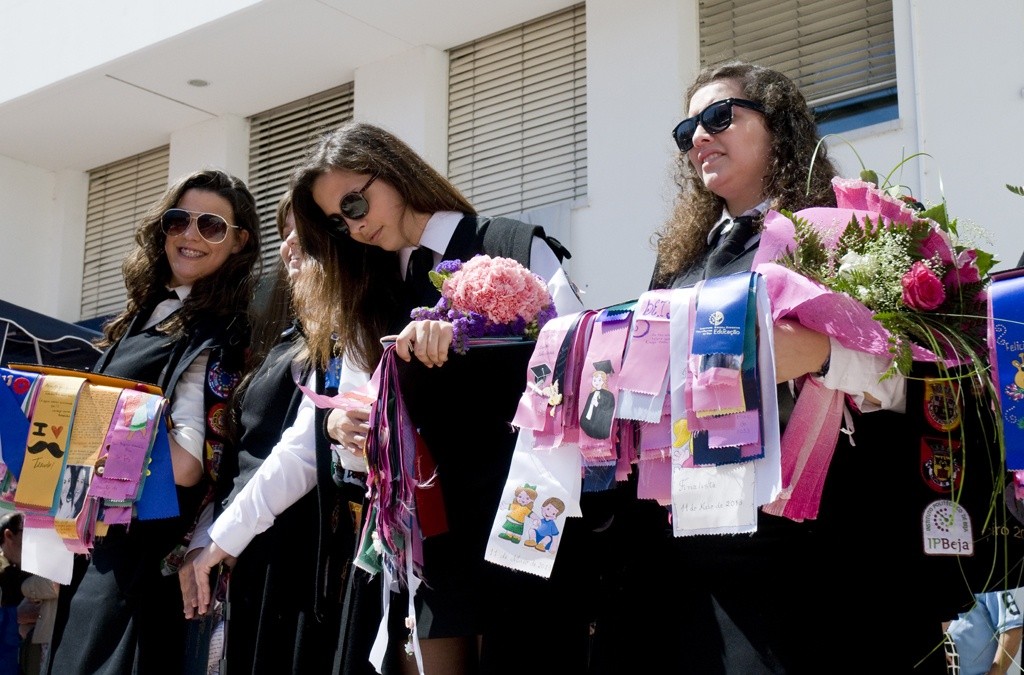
[410,253,558,354]
[752,132,1024,675]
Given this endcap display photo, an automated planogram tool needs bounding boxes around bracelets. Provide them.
[815,356,830,377]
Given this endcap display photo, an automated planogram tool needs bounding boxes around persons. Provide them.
[0,59,1024,675]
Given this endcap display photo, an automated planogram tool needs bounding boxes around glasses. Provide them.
[160,208,242,245]
[321,171,381,244]
[671,98,765,152]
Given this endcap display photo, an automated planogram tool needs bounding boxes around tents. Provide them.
[0,300,106,373]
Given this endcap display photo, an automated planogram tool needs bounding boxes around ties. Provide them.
[409,245,433,294]
[128,286,180,336]
[704,216,761,281]
